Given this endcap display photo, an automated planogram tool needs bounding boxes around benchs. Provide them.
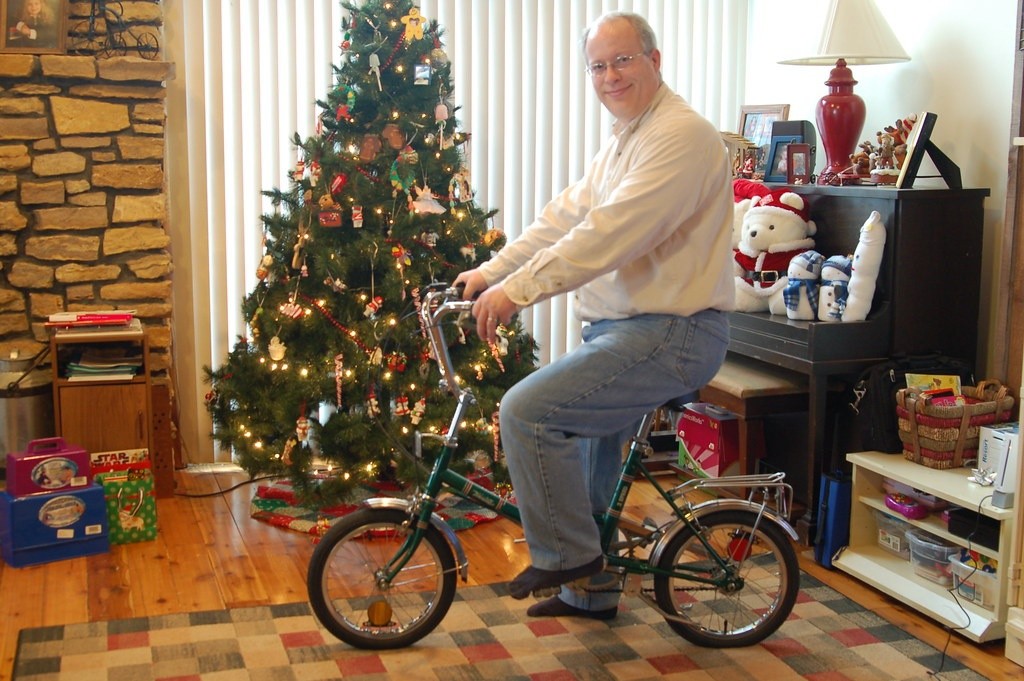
[636,354,843,545]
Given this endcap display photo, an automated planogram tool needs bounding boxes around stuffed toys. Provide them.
[732,179,886,323]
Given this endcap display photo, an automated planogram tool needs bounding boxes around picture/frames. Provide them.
[896,111,938,189]
[764,136,804,182]
[737,104,790,180]
[0,0,69,55]
[788,143,810,184]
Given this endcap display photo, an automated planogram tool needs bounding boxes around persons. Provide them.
[778,145,787,174]
[746,116,756,137]
[452,10,734,620]
[794,155,804,175]
[849,112,917,174]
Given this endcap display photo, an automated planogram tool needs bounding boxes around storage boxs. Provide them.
[677,403,767,498]
[95,468,158,545]
[978,421,1019,476]
[872,510,995,611]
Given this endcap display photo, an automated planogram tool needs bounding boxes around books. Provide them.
[45,309,137,326]
[65,347,142,383]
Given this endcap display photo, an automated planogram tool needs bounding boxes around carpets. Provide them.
[249,471,516,537]
[9,552,992,681]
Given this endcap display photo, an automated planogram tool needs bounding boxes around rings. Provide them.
[487,318,498,322]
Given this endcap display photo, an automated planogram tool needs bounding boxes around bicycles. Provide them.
[307,284,802,650]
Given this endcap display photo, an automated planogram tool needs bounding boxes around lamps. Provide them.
[775,0,912,186]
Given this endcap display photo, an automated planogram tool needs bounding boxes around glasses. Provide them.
[586,52,643,76]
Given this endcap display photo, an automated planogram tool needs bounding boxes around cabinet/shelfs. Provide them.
[49,321,155,482]
[829,450,1013,647]
